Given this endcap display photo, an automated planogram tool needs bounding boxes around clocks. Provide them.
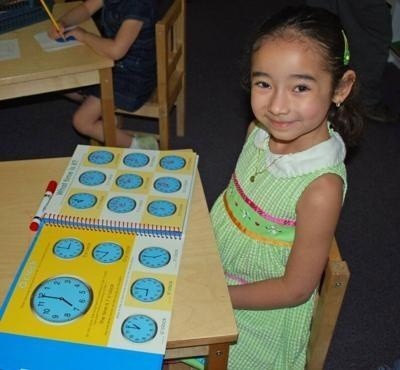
[27,148,188,345]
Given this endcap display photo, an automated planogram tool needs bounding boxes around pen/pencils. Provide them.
[29,180,57,232]
[40,0,67,42]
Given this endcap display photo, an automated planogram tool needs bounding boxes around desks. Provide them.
[0,0,116,147]
[0,149,242,370]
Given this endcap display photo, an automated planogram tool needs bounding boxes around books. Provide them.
[0,142,201,370]
[32,25,83,52]
[0,39,22,60]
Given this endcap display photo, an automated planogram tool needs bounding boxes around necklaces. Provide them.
[250,142,276,186]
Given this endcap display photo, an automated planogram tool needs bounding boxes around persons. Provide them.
[308,1,400,127]
[210,6,371,370]
[48,0,176,149]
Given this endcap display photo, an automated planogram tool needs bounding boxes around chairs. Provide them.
[169,233,351,370]
[89,0,185,150]
